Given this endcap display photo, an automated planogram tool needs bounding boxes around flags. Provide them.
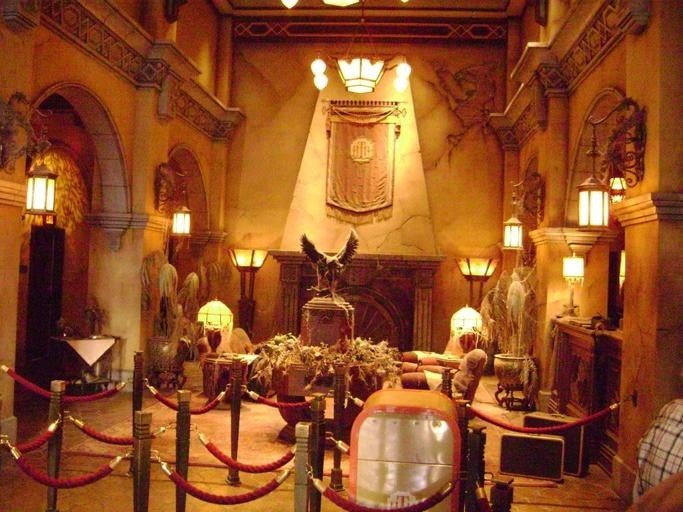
[324,99,401,226]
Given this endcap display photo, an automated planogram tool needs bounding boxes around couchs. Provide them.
[197,329,261,359]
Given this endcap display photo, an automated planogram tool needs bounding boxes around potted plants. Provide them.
[246,330,404,431]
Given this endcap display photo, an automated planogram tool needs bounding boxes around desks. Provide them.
[51,331,119,399]
[202,361,246,403]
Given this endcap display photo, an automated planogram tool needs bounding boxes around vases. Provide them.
[148,334,183,372]
[493,351,536,387]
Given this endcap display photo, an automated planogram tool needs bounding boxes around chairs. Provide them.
[400,348,487,403]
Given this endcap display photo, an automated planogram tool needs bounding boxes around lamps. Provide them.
[311,0,412,94]
[606,165,628,204]
[198,297,233,358]
[0,93,60,227]
[503,170,547,251]
[455,256,499,316]
[556,251,586,319]
[575,96,647,236]
[155,162,192,238]
[451,302,484,354]
[282,0,410,9]
[227,247,268,344]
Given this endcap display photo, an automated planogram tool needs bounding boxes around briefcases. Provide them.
[524,409,590,478]
[500,431,565,482]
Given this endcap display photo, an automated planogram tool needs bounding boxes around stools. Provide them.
[494,384,534,410]
